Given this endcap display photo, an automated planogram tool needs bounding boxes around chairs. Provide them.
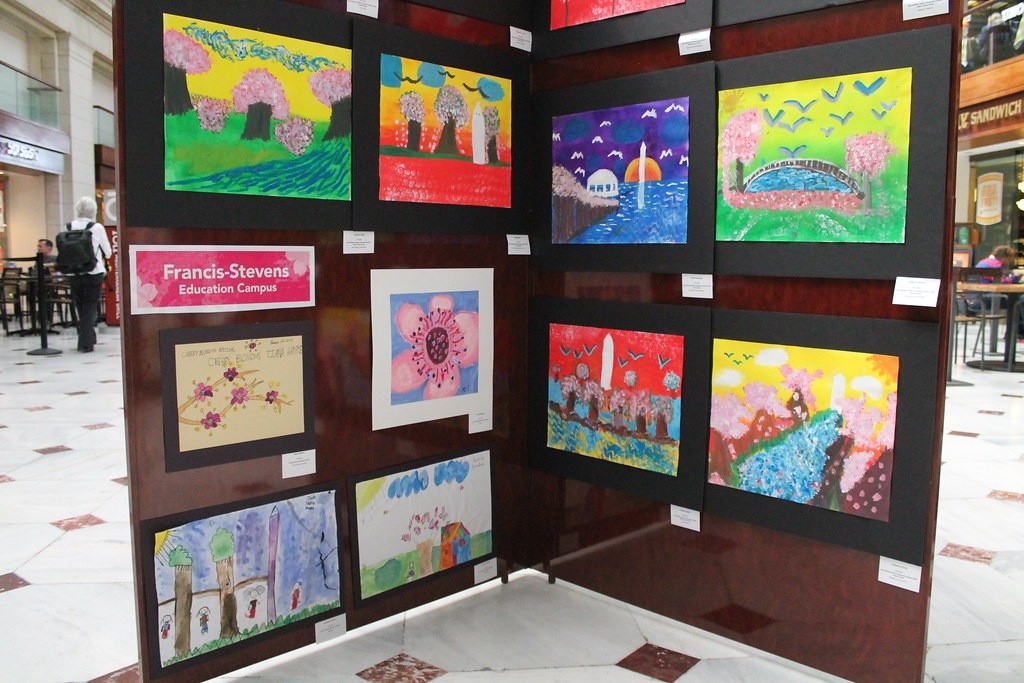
[1,267,76,337]
[952,267,1023,368]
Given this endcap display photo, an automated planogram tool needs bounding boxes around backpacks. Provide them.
[55,221,100,273]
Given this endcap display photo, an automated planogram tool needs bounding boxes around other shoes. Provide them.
[77,343,94,351]
[1005,331,1024,340]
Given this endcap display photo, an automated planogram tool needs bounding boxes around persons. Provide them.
[33,239,58,328]
[964,247,1024,343]
[61,197,112,351]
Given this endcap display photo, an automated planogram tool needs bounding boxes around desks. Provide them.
[957,283,1024,373]
